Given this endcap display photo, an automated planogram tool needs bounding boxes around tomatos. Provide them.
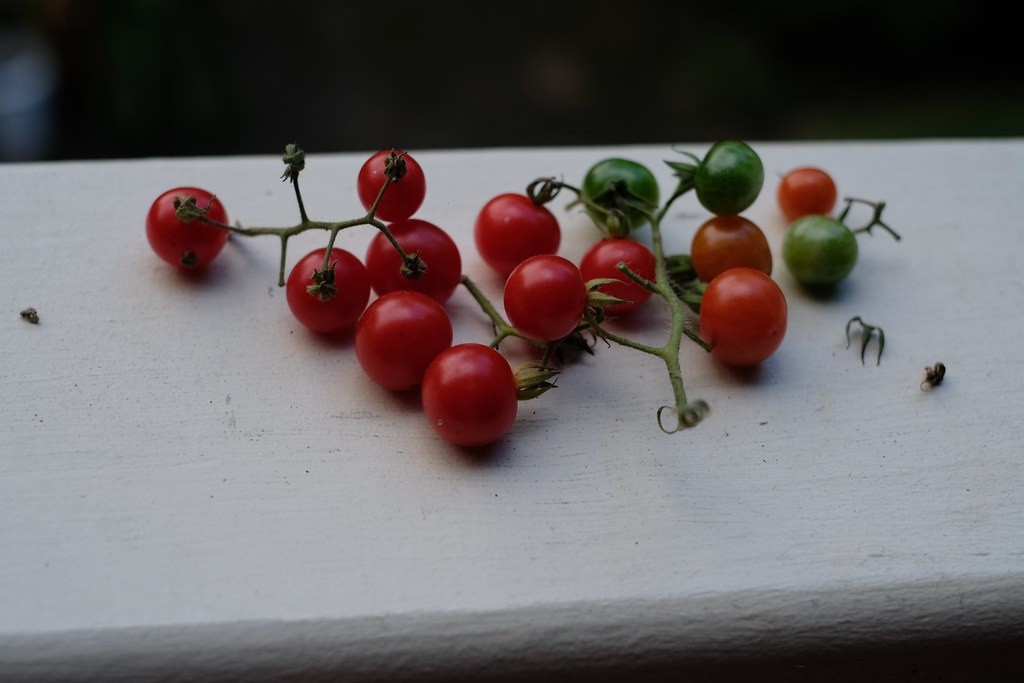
[285,140,860,448]
[146,186,229,271]
